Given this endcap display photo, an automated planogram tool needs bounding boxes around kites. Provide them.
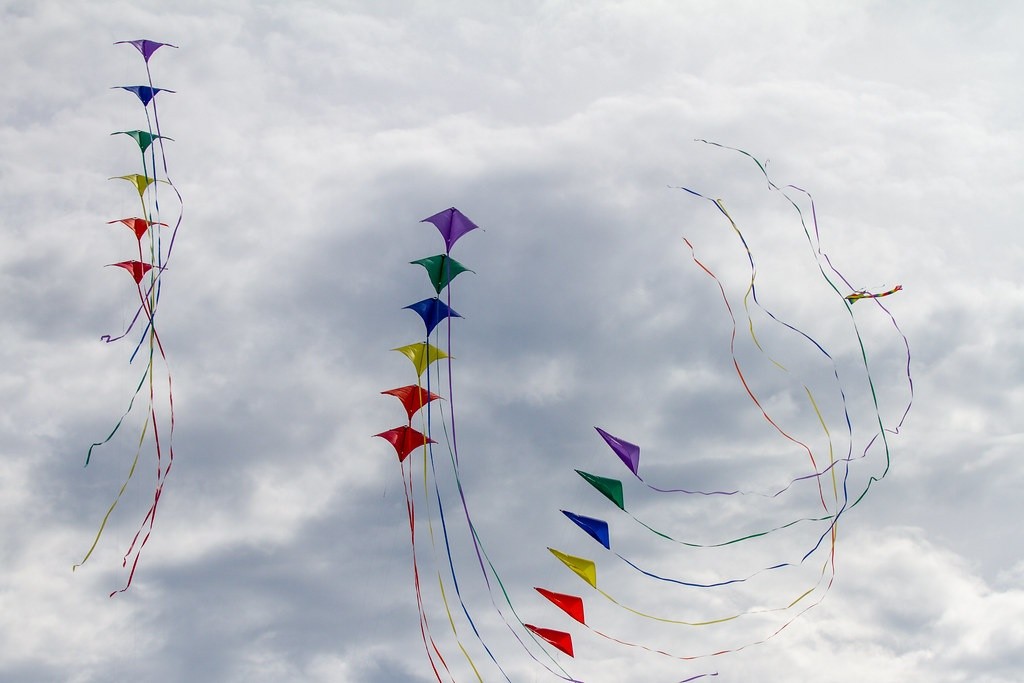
[71,39,183,597]
[372,138,913,683]
